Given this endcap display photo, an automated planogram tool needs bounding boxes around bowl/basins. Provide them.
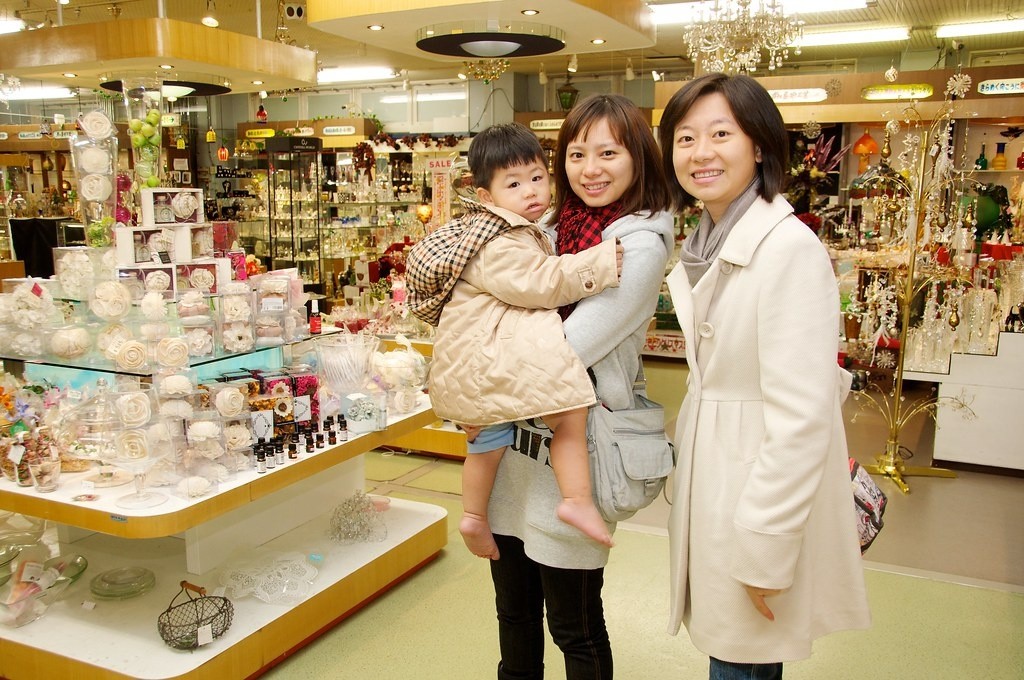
[371,355,434,392]
[0,542,20,586]
[0,576,72,628]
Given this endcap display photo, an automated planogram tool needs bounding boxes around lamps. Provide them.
[532,53,637,86]
[825,74,995,496]
[412,18,566,59]
[555,61,579,113]
[94,70,236,106]
[682,1,807,77]
[201,1,221,28]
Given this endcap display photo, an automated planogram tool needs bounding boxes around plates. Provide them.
[89,566,156,601]
[42,553,88,585]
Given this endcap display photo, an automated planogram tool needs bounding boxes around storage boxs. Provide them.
[313,115,378,137]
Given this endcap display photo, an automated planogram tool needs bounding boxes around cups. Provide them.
[28,457,61,493]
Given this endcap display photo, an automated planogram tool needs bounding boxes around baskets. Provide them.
[158,596,234,650]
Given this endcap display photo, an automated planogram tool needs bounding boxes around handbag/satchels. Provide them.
[849,459,887,554]
[585,393,676,523]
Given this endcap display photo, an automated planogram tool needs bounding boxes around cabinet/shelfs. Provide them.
[0,121,187,259]
[0,327,451,680]
[231,146,462,305]
[945,119,1024,242]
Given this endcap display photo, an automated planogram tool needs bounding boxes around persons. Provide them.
[462,94,676,680]
[406,121,624,560]
[658,73,868,680]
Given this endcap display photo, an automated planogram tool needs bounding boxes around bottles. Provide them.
[309,302,322,334]
[253,434,285,473]
[288,413,348,459]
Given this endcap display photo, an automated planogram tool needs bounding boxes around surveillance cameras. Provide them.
[952,40,967,50]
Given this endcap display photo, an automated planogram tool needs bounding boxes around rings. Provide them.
[758,594,764,597]
